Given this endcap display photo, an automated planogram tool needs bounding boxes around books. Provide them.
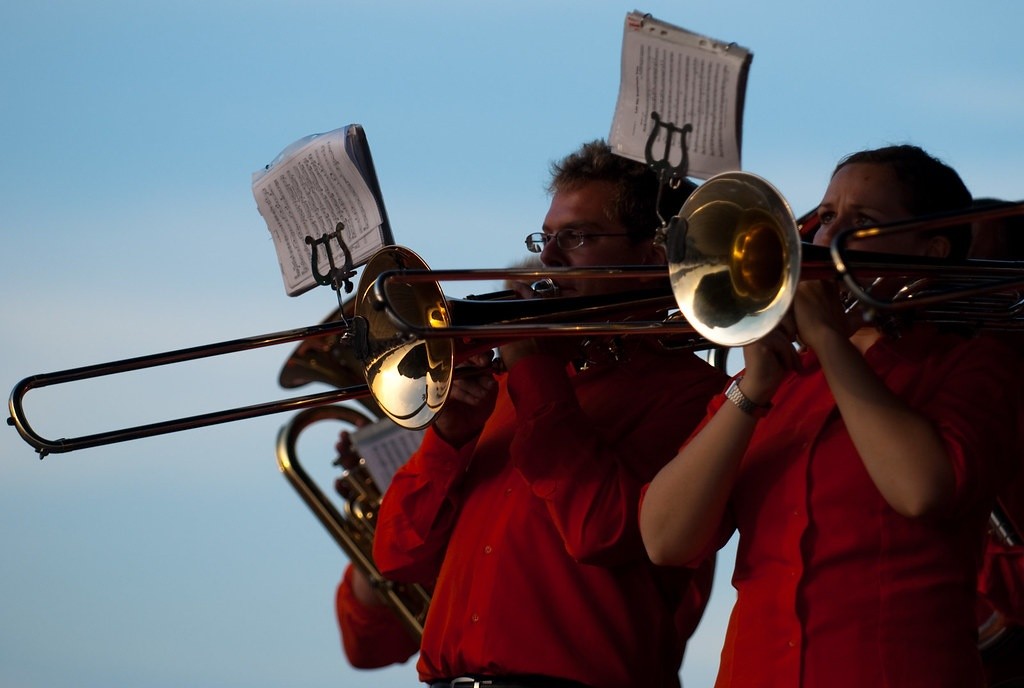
[251,123,395,297]
[606,10,754,180]
[349,417,422,493]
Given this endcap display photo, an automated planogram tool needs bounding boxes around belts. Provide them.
[430,673,585,688]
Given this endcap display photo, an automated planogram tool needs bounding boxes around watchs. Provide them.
[724,375,773,417]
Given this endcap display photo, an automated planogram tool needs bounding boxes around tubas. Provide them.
[274,293,432,636]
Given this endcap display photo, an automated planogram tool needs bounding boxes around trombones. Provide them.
[825,169,1020,349]
[372,169,838,352]
[5,242,566,461]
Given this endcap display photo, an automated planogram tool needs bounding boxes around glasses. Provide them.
[525,229,629,250]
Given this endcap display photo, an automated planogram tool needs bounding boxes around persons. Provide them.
[336,137,731,688]
[636,145,1024,688]
